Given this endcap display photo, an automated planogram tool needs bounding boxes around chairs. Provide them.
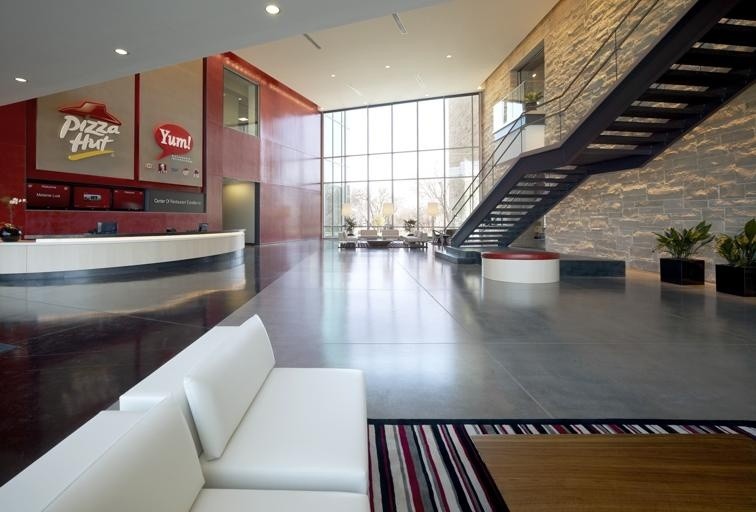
[338,229,428,248]
[432,230,443,243]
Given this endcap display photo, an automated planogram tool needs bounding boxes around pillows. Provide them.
[44,392,205,510]
[183,313,275,460]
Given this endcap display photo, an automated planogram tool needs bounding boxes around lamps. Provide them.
[341,203,352,230]
[427,202,440,244]
[382,202,393,229]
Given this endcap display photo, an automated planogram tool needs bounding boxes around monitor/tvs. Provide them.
[96,222,118,234]
[199,223,208,232]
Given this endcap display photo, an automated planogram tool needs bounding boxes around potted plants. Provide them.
[714,219,756,297]
[652,219,714,286]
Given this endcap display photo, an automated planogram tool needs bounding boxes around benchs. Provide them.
[481,250,560,284]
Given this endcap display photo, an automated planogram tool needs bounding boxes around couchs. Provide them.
[0,325,369,512]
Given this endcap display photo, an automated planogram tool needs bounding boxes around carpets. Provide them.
[368,419,756,512]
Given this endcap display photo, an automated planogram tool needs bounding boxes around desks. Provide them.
[466,433,756,509]
[436,235,450,245]
